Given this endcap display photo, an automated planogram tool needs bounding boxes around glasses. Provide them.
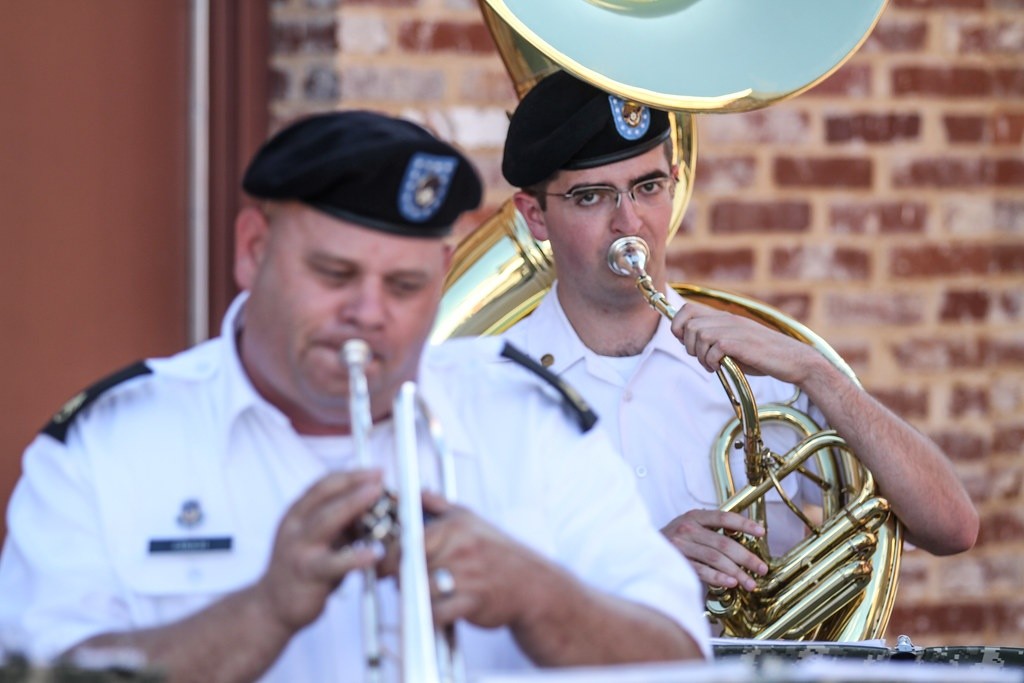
[539,177,678,215]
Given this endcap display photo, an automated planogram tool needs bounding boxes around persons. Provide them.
[499,62,981,592]
[1,109,719,683]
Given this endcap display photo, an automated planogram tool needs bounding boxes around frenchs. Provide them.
[422,2,906,641]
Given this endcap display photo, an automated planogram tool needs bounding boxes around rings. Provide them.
[434,567,456,596]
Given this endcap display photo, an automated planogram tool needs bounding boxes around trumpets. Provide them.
[340,335,469,681]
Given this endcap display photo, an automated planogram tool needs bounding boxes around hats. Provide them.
[243,112,482,238]
[501,68,672,189]
